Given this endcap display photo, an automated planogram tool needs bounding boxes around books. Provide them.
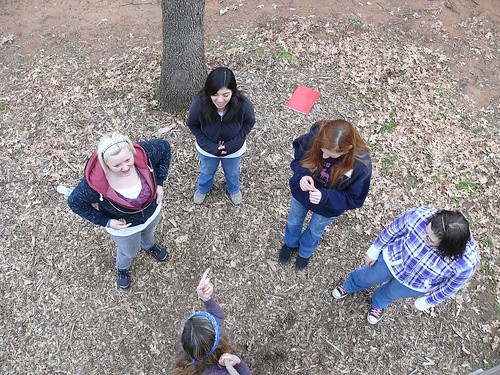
[286,85,320,115]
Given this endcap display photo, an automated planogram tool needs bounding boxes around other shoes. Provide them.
[229,191,242,205]
[193,189,209,204]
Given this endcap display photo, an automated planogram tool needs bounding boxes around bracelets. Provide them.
[107,219,111,228]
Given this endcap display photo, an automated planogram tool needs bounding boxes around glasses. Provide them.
[424,221,439,248]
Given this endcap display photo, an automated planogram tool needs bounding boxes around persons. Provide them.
[175,268,252,375]
[280,118,373,269]
[186,67,255,205]
[333,206,480,325]
[68,132,171,288]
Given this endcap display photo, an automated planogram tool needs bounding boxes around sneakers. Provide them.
[141,244,168,261]
[279,244,311,270]
[331,282,349,299]
[366,306,385,324]
[117,268,130,288]
